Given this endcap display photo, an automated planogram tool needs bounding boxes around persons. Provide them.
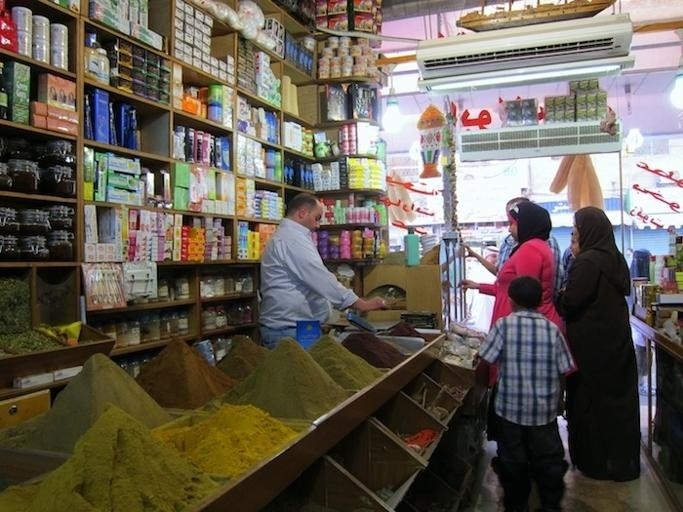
[255,193,390,350]
[477,275,575,511]
[552,206,640,481]
[456,197,566,465]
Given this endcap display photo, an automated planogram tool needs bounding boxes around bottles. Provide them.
[103,271,253,347]
[84,40,111,86]
[404,227,419,267]
[118,358,155,380]
[0,63,8,119]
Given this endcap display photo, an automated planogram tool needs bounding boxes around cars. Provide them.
[482,235,497,246]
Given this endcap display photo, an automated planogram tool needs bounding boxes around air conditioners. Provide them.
[416,13,634,79]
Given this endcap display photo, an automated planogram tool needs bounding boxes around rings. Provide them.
[382,300,385,303]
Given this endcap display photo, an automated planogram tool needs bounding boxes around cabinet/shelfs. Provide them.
[0,0,491,511]
[629,311,682,510]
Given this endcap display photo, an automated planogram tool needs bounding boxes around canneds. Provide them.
[199,271,253,298]
[318,36,379,79]
[94,310,188,349]
[158,274,189,301]
[11,6,68,71]
